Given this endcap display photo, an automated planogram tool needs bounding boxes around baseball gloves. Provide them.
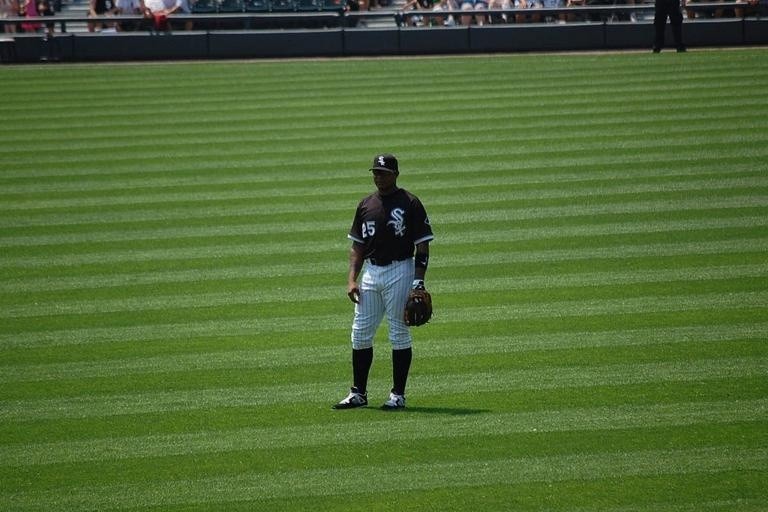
[404,289,432,326]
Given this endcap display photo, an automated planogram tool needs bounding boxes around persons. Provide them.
[336,155,433,409]
[650,1,686,54]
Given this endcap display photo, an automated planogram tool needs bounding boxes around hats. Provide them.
[369,153,399,174]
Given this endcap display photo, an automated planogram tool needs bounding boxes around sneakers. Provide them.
[380,392,405,411]
[331,386,368,410]
